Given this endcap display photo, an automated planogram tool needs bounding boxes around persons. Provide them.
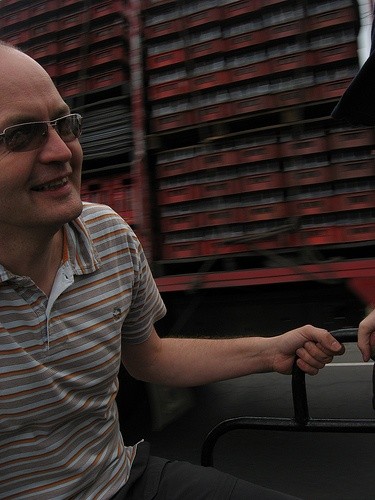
[357,310,375,360]
[0,40,346,499]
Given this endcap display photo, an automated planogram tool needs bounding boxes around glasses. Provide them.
[0,114,84,153]
